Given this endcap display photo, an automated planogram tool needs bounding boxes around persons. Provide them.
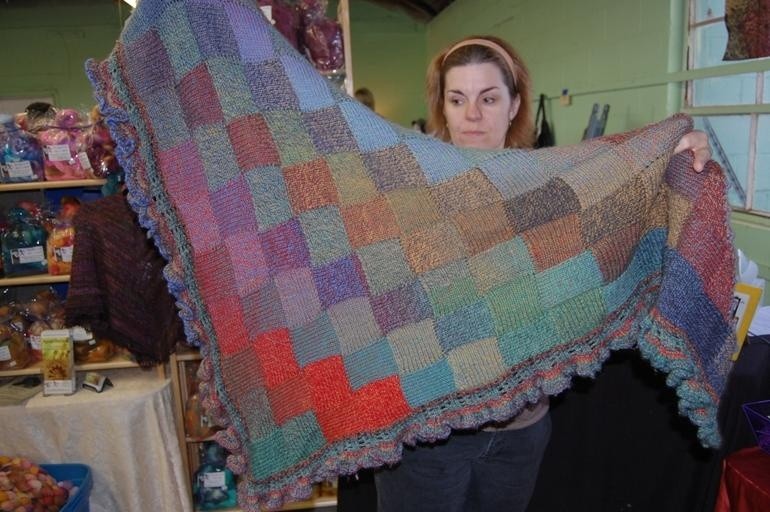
[65,180,187,373]
[410,119,427,134]
[355,86,375,112]
[369,32,712,511]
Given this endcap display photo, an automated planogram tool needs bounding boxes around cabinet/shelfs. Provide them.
[2,1,354,510]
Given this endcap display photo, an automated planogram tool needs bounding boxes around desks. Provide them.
[718,446,770,500]
